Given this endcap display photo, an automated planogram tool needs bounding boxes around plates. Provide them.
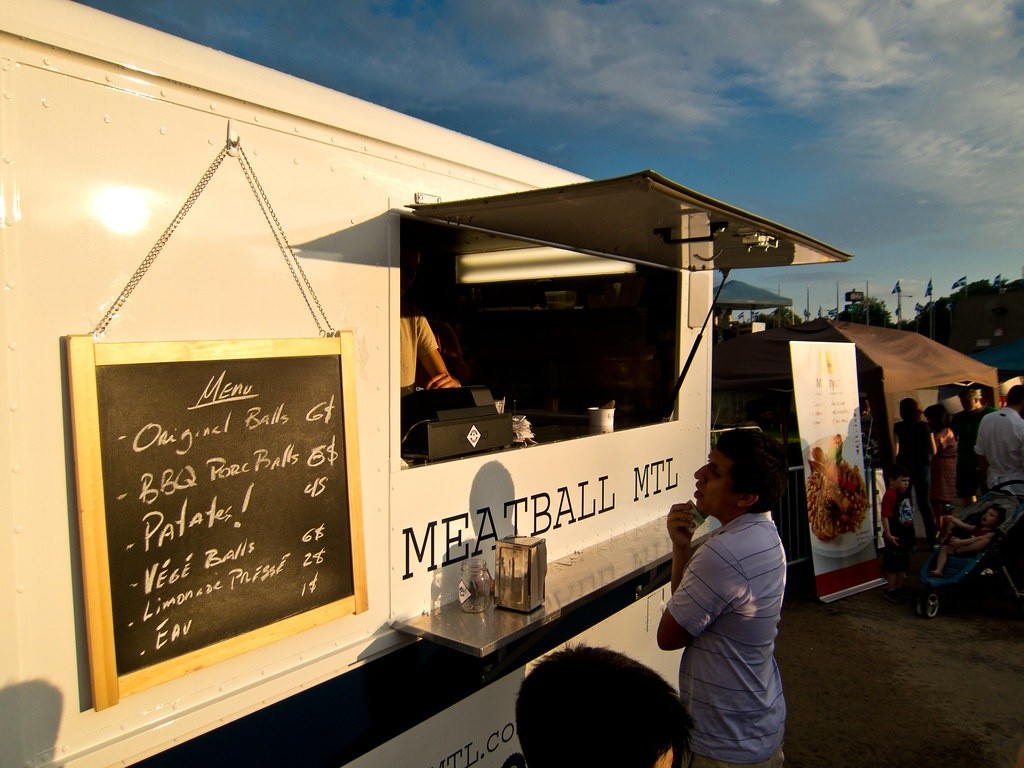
[812,541,869,559]
[809,510,870,552]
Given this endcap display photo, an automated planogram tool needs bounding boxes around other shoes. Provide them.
[882,590,906,604]
[911,545,917,553]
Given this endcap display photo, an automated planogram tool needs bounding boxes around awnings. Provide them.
[406,170,855,270]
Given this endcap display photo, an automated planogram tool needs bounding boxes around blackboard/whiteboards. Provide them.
[63,330,369,714]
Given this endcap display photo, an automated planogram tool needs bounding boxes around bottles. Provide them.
[459,560,491,613]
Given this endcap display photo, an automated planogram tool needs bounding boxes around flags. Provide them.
[952,276,967,289]
[946,303,953,311]
[915,303,923,312]
[895,305,901,316]
[993,275,1002,285]
[891,281,902,294]
[925,279,932,297]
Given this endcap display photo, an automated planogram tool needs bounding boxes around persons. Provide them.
[657,428,788,768]
[857,384,1024,601]
[515,643,696,768]
[400,238,462,396]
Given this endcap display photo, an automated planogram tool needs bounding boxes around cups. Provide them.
[588,406,615,434]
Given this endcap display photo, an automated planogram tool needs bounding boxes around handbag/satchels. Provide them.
[928,431,937,455]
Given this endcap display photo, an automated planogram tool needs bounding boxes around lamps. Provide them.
[455,246,641,284]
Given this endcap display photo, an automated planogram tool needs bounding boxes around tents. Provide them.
[712,318,1000,464]
[712,279,793,310]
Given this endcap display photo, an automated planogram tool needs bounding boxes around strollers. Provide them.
[920,479,1024,620]
